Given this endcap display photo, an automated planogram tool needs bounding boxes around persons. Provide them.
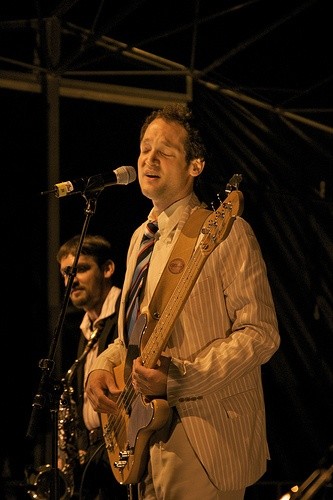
[48,234,136,500]
[81,104,281,500]
[31,407,107,496]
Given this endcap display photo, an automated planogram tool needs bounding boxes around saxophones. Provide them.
[28,321,102,500]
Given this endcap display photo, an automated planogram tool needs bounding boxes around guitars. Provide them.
[99,173,245,484]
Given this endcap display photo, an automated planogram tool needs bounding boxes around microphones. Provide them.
[40,164,138,199]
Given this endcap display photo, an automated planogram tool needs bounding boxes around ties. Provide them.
[123,221,159,350]
